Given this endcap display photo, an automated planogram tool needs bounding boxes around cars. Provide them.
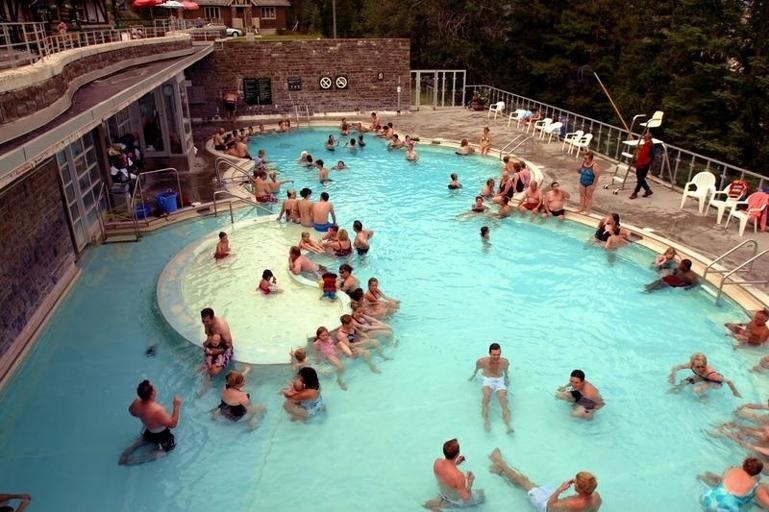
[196,22,243,37]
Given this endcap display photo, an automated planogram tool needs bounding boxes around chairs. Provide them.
[639,110,664,134]
[679,172,717,214]
[704,180,747,225]
[487,101,593,161]
[724,192,767,236]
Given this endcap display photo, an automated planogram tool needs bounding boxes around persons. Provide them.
[57,22,67,36]
[0,494,31,512]
[668,309,769,512]
[196,16,204,28]
[556,370,605,421]
[423,439,485,512]
[223,91,237,124]
[489,448,602,512]
[467,343,515,434]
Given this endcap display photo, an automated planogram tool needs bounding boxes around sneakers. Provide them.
[629,192,638,199]
[642,190,653,198]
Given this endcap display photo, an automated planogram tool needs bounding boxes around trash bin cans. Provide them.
[155,191,178,214]
[135,206,151,220]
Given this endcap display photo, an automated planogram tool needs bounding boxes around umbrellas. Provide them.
[130,0,167,20]
[154,0,182,20]
[177,0,199,19]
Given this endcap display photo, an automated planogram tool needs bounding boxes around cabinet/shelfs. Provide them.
[105,132,147,206]
